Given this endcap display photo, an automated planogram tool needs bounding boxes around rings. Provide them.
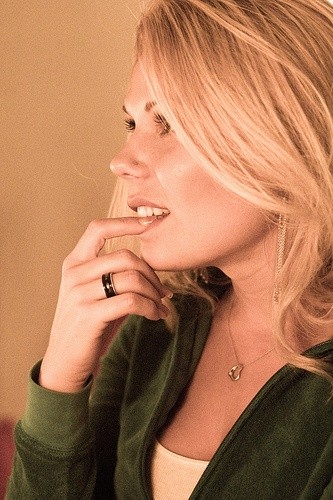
[101,270,116,298]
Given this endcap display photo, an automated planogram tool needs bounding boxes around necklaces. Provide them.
[225,286,326,382]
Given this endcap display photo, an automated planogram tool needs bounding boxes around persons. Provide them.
[0,0,333,500]
